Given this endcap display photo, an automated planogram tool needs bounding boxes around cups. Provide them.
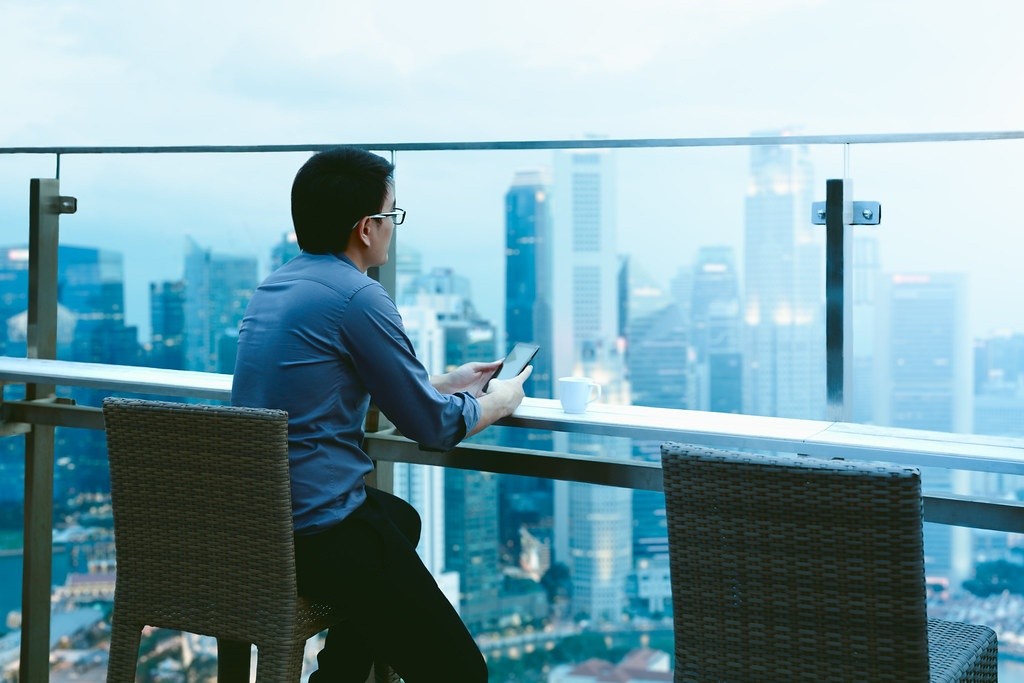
[558,376,602,414]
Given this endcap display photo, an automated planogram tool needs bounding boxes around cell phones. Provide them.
[482,343,540,393]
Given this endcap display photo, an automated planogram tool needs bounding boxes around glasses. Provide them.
[351,207,406,229]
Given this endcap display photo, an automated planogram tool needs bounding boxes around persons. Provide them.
[226,147,532,682]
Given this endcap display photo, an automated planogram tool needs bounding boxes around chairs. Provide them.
[102,396,400,683]
[658,440,998,683]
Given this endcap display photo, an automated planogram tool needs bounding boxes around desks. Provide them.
[0,372,1024,683]
